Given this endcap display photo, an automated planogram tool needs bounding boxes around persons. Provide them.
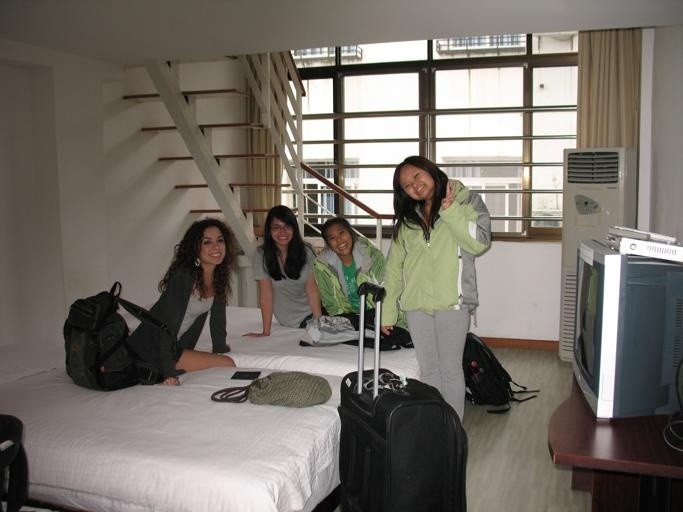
[131,218,236,386]
[314,217,410,332]
[373,155,491,424]
[242,205,321,337]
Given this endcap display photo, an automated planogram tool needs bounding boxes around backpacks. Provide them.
[63,282,176,392]
[462,332,540,414]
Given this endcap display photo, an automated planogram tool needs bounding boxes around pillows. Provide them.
[0,351,55,384]
[119,309,149,336]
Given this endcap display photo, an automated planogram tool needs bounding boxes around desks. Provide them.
[546,372,681,511]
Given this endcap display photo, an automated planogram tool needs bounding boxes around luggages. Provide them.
[337,283,466,511]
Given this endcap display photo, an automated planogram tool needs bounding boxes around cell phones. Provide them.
[230,371,261,379]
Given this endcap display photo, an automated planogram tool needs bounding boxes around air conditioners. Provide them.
[557,147,638,362]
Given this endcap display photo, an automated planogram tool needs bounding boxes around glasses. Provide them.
[269,225,295,230]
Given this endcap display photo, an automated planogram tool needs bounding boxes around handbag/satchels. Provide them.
[211,373,332,408]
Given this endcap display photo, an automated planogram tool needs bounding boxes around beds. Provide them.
[128,306,421,381]
[0,364,344,512]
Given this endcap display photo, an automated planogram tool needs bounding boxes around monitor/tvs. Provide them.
[571,238,683,422]
